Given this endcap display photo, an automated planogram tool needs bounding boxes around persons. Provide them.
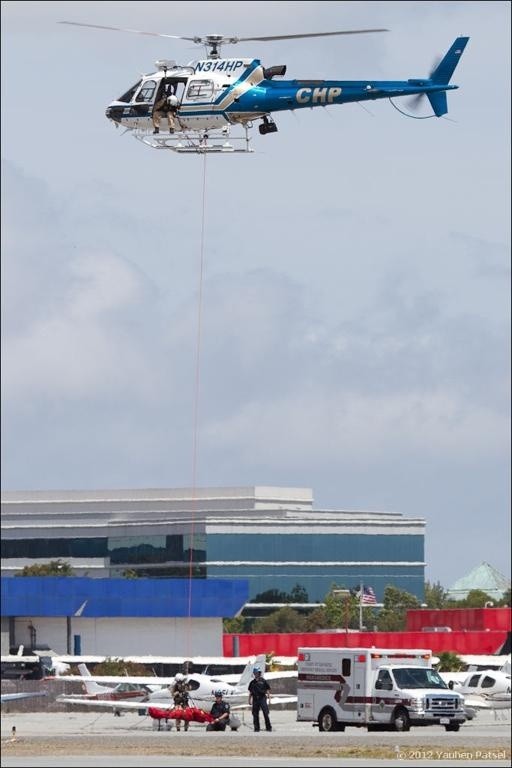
[169,672,193,731]
[163,90,179,118]
[246,667,274,731]
[151,89,179,135]
[205,690,232,731]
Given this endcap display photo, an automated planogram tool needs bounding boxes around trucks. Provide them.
[294,646,475,733]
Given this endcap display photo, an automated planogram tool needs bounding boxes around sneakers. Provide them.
[253,729,273,732]
[177,728,187,731]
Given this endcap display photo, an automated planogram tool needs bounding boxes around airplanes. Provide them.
[59,20,470,154]
[452,668,510,712]
[54,654,298,734]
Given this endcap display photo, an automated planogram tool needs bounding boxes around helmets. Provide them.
[214,689,222,697]
[253,667,261,673]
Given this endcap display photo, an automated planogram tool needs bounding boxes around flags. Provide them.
[362,584,377,604]
